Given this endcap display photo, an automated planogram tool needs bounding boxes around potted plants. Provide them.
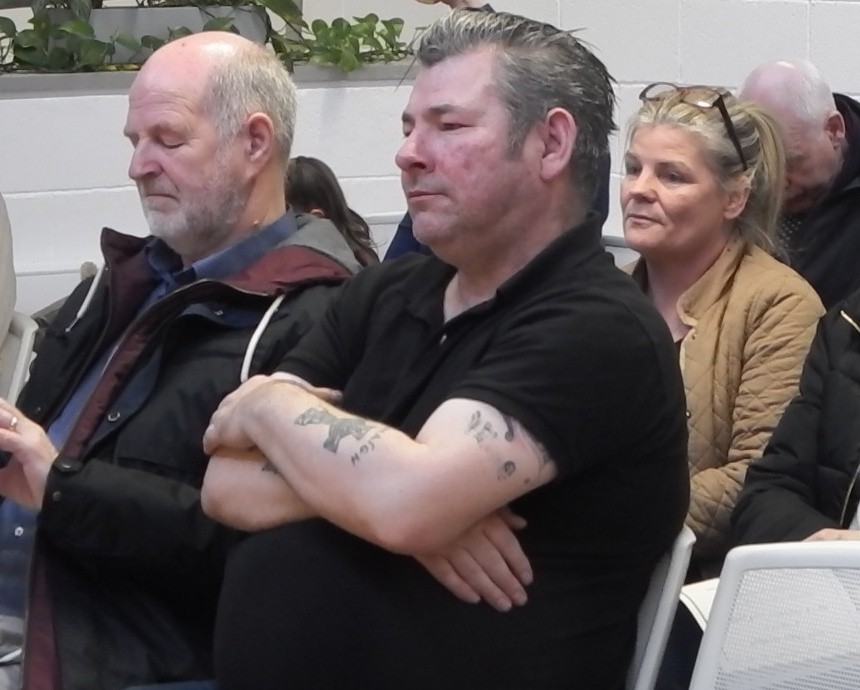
[0,0,430,76]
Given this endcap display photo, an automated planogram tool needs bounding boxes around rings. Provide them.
[8,416,19,431]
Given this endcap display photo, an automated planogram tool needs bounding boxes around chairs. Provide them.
[688,542,859,690]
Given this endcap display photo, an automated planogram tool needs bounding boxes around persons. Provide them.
[729,290,860,558]
[284,157,382,269]
[0,32,363,690]
[383,125,611,260]
[0,191,16,347]
[628,59,860,309]
[616,78,828,690]
[199,4,690,690]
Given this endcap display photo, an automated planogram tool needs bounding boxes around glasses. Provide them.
[639,81,748,173]
